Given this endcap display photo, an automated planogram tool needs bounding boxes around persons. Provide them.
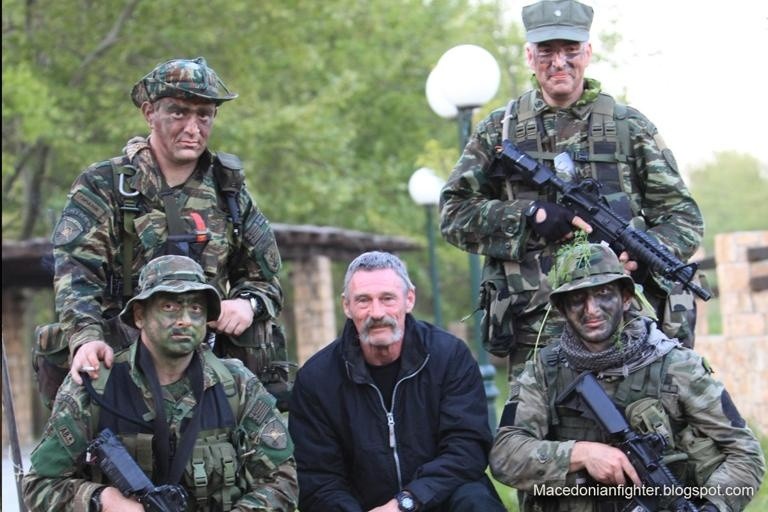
[436,0,706,367]
[20,252,300,512]
[487,242,766,512]
[286,248,510,512]
[33,53,293,426]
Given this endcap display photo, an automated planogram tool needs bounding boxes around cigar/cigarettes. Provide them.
[79,366,96,372]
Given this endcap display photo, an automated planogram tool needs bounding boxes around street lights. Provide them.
[425,43,498,438]
[409,167,445,330]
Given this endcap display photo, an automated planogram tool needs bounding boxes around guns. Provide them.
[85,428,188,511]
[558,370,701,512]
[493,140,710,302]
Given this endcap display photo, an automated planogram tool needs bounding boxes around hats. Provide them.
[119,256,221,327]
[130,57,238,110]
[522,0,595,43]
[545,243,635,316]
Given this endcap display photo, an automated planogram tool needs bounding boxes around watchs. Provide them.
[90,485,108,512]
[392,490,419,512]
[241,292,264,318]
